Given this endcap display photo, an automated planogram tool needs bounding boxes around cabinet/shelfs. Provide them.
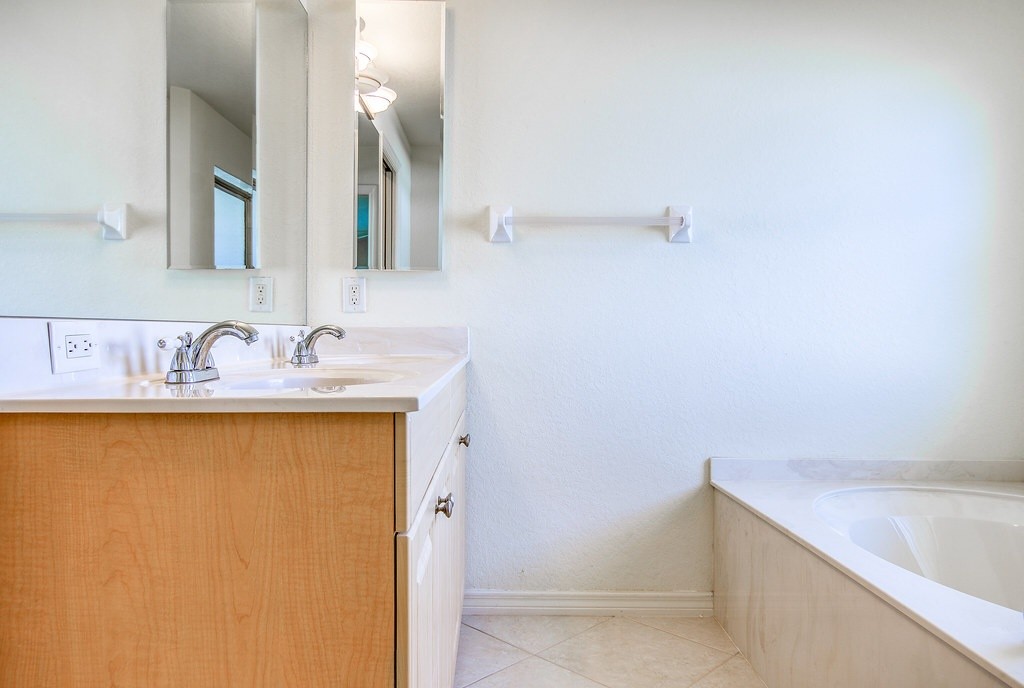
[0,366,470,687]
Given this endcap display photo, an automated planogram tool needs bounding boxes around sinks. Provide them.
[203,371,395,391]
[317,357,441,363]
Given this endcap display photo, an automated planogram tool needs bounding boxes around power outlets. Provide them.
[343,276,366,312]
[249,276,273,312]
[48,320,100,374]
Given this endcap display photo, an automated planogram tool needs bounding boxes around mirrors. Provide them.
[353,0,446,272]
[166,0,257,270]
[0,0,308,326]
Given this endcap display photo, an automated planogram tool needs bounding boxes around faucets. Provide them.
[158,320,259,384]
[287,323,346,365]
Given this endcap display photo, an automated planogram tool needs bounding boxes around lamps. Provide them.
[354,14,398,120]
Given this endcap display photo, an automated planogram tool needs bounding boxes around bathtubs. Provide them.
[710,479,1024,687]
